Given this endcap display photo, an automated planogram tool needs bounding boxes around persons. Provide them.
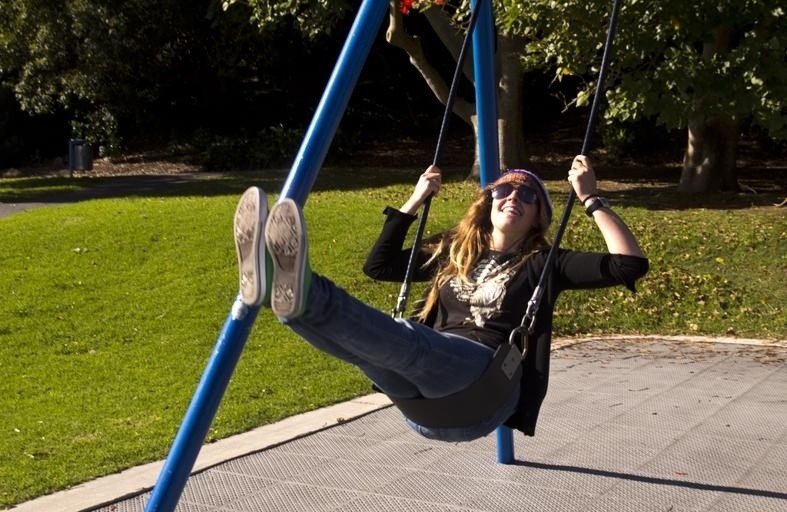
[229,151,652,445]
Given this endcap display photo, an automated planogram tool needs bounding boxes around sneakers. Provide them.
[264,198,312,324]
[232,186,273,308]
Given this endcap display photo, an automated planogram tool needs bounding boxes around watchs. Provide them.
[583,197,609,219]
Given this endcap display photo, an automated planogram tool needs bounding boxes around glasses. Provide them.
[491,183,540,205]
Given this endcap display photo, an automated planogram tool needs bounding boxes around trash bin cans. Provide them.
[69,138,88,177]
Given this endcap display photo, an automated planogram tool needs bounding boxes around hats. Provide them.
[501,168,552,227]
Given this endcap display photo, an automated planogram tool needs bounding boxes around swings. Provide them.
[394,1,622,428]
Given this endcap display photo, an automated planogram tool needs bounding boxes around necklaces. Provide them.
[486,233,525,263]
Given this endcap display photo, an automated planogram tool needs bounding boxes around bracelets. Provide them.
[580,193,600,206]
